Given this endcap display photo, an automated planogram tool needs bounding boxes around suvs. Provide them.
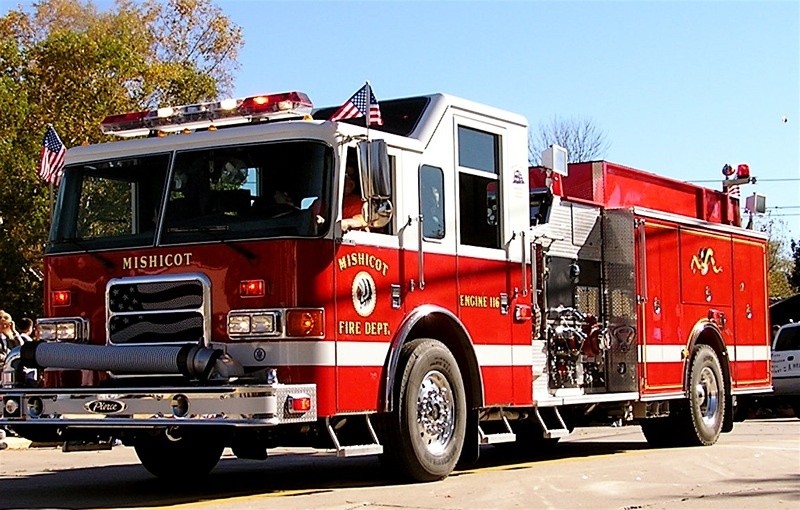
[732,293,800,420]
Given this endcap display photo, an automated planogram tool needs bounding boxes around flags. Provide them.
[329,81,385,126]
[38,123,67,187]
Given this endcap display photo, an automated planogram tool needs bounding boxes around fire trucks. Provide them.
[1,83,800,488]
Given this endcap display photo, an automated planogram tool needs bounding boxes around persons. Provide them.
[301,158,373,233]
[0,309,39,438]
